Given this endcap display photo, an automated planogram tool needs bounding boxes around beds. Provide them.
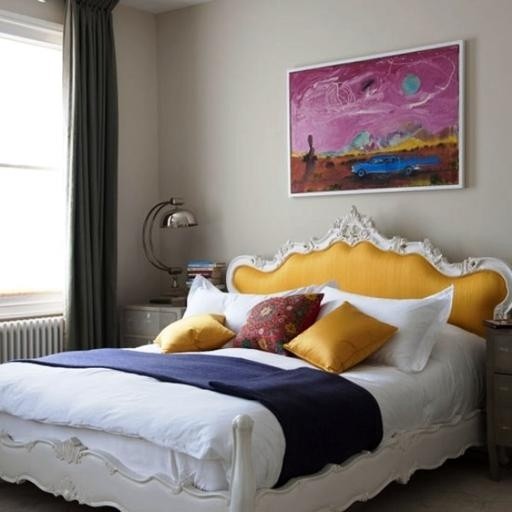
[1,204,511,512]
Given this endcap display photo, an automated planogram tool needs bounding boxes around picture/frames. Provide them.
[285,40,467,201]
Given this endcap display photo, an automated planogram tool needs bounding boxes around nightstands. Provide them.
[116,304,189,347]
[479,316,512,483]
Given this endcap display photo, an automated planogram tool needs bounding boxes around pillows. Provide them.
[311,283,457,373]
[181,271,339,344]
[154,311,240,351]
[231,291,326,357]
[281,300,399,376]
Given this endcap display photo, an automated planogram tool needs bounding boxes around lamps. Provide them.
[139,196,202,305]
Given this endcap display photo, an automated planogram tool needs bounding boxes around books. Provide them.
[483,316,512,328]
[182,257,225,294]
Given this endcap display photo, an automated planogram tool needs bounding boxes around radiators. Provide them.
[0,312,69,363]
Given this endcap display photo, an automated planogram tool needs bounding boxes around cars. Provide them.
[350,152,442,178]
